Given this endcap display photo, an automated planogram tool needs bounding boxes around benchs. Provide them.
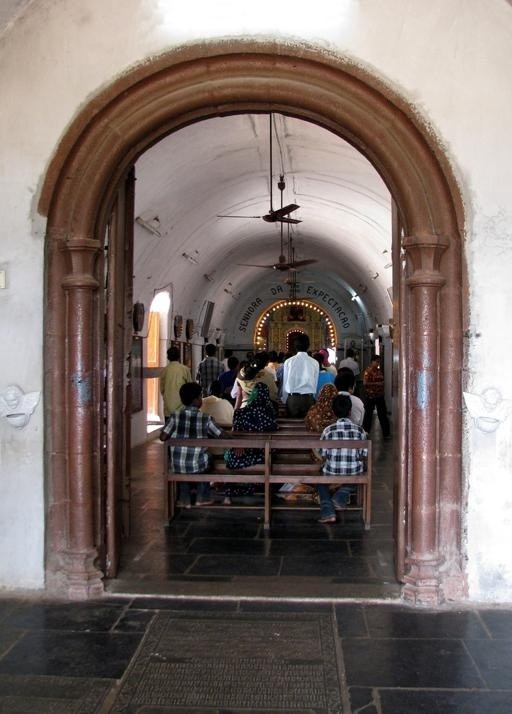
[172,431,367,495]
[272,417,313,462]
[164,438,372,530]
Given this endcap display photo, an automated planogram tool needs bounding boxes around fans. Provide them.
[266,214,318,284]
[236,175,319,272]
[271,230,317,301]
[215,111,303,224]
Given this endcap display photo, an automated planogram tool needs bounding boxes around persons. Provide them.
[218,356,240,384]
[220,349,234,372]
[334,367,365,428]
[312,394,369,476]
[281,334,320,421]
[231,350,285,399]
[312,352,335,400]
[159,346,192,459]
[338,348,360,377]
[319,348,337,375]
[304,383,338,463]
[160,382,229,509]
[196,344,225,397]
[363,355,383,427]
[244,351,256,362]
[225,382,279,505]
[200,380,234,428]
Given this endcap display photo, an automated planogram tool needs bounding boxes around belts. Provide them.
[288,392,313,397]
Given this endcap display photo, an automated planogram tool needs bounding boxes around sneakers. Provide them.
[385,435,392,439]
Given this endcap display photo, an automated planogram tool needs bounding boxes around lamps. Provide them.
[384,262,393,270]
[182,252,199,265]
[204,274,214,282]
[136,217,161,238]
[371,273,379,281]
